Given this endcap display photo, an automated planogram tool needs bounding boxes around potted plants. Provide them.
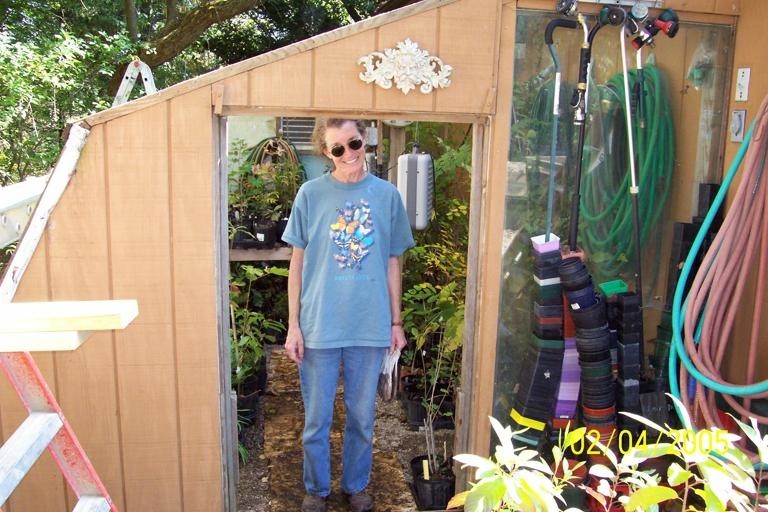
[231,292,285,426]
[401,283,468,512]
[250,163,281,250]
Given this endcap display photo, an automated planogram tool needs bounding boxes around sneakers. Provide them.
[301,494,327,512]
[348,492,373,512]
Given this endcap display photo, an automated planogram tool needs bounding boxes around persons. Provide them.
[281,117,416,512]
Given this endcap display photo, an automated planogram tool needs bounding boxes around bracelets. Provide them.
[392,321,404,327]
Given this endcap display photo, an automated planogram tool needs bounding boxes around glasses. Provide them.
[329,138,362,157]
